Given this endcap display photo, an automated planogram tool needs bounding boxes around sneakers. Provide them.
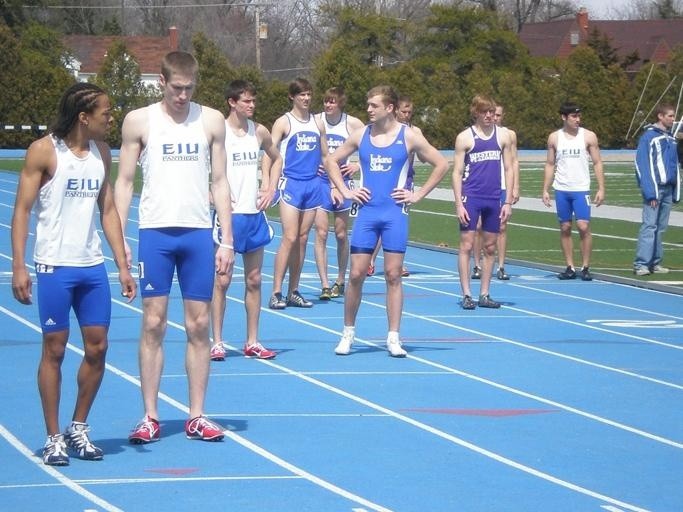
[185,416,224,440]
[335,336,352,354]
[463,295,500,309]
[210,345,224,360]
[244,342,275,359]
[269,289,312,308]
[636,265,669,275]
[43,421,103,465]
[320,283,344,300]
[497,267,509,280]
[559,265,576,280]
[581,266,591,280]
[129,416,159,443]
[471,266,482,278]
[387,340,407,357]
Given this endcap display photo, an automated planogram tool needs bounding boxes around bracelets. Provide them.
[217,242,233,251]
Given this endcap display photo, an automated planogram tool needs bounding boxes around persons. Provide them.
[211,78,449,360]
[542,99,606,281]
[112,50,235,444]
[633,104,681,275]
[10,82,137,467]
[451,92,520,310]
